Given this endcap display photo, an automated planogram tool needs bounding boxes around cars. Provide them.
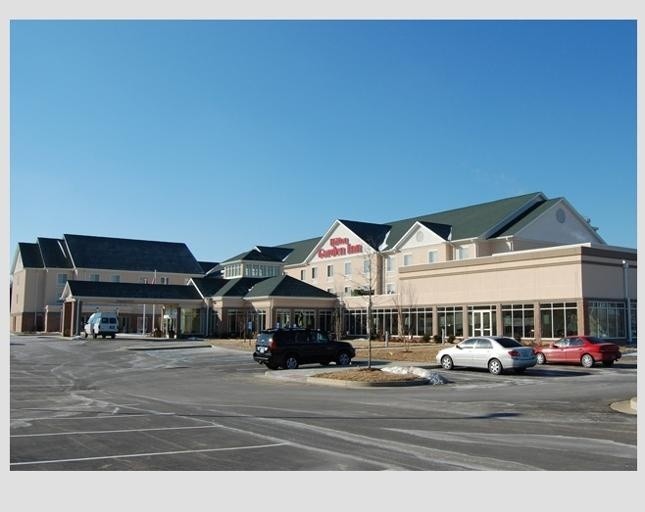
[435,335,537,375]
[534,335,621,368]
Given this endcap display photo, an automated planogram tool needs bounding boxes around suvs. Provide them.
[253,326,356,369]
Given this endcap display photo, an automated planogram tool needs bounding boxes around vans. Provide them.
[83,311,120,339]
